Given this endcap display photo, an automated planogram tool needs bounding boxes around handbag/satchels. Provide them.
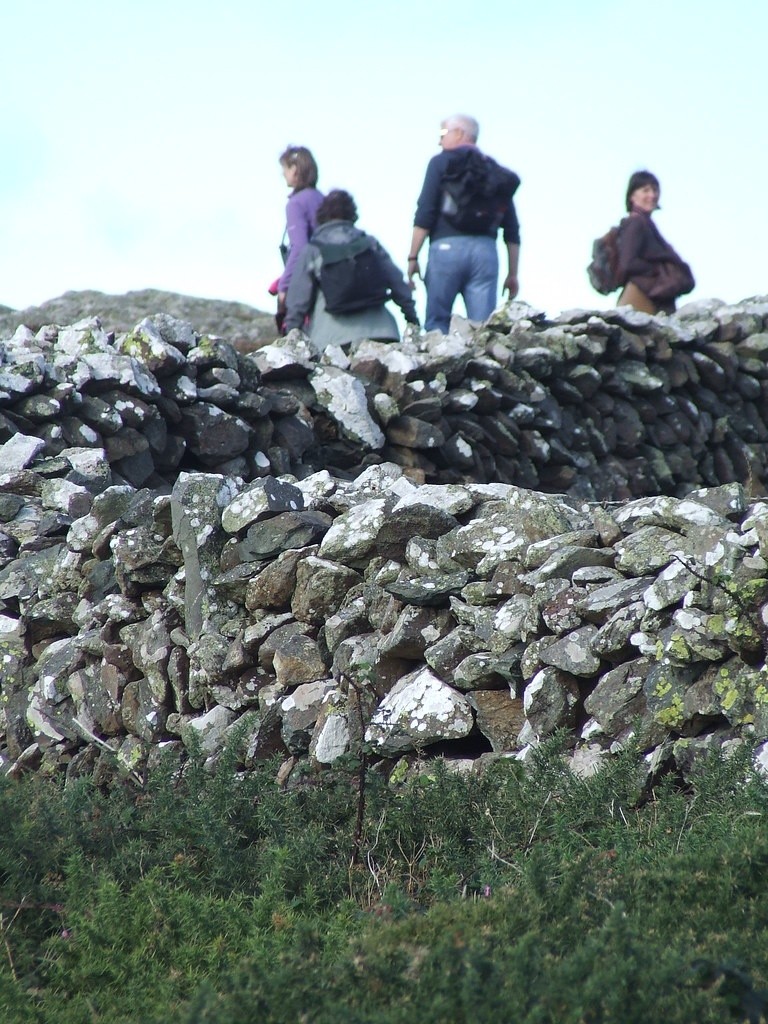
[648,255,695,299]
[441,147,520,235]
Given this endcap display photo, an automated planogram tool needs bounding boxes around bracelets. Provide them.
[406,257,417,261]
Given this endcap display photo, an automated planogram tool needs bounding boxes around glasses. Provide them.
[440,128,452,138]
[289,149,298,165]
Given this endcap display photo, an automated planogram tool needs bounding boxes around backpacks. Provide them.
[588,220,628,295]
[310,232,388,315]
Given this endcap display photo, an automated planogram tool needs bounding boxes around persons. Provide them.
[586,169,695,315]
[269,144,423,358]
[407,115,520,334]
